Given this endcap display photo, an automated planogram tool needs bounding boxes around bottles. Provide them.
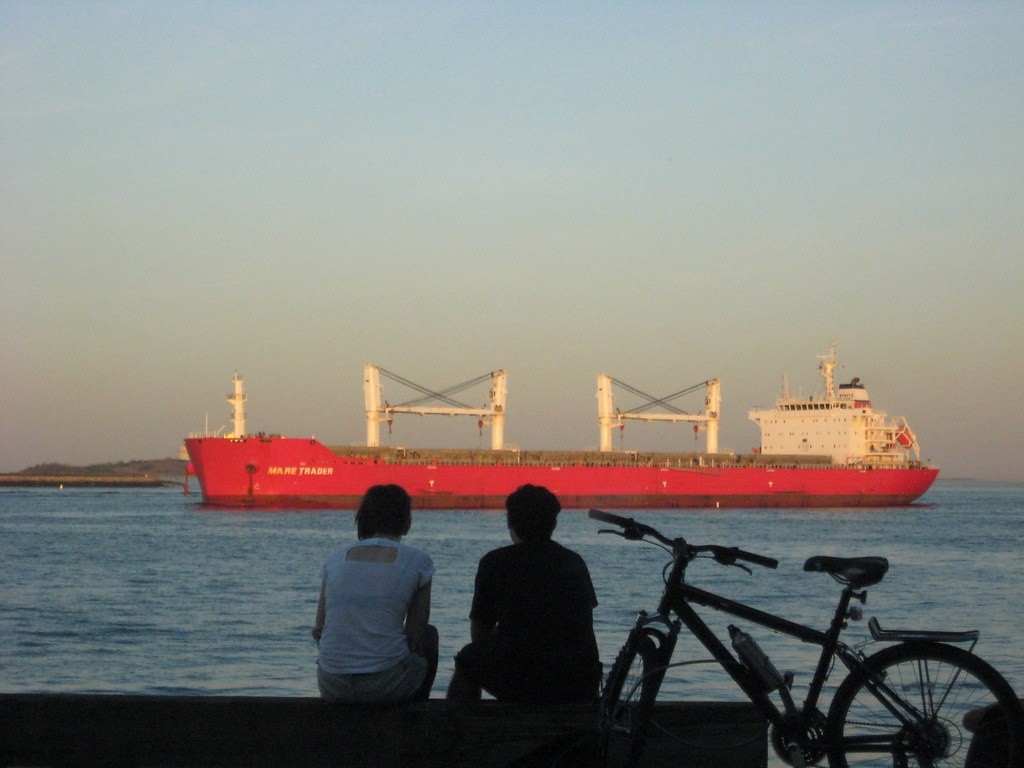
[726,624,782,694]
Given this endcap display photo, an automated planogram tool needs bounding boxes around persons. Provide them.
[446,484,603,703]
[316,484,439,706]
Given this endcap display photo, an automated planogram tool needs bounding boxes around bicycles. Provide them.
[589,509,1019,768]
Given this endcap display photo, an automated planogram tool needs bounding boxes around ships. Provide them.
[183,340,941,510]
